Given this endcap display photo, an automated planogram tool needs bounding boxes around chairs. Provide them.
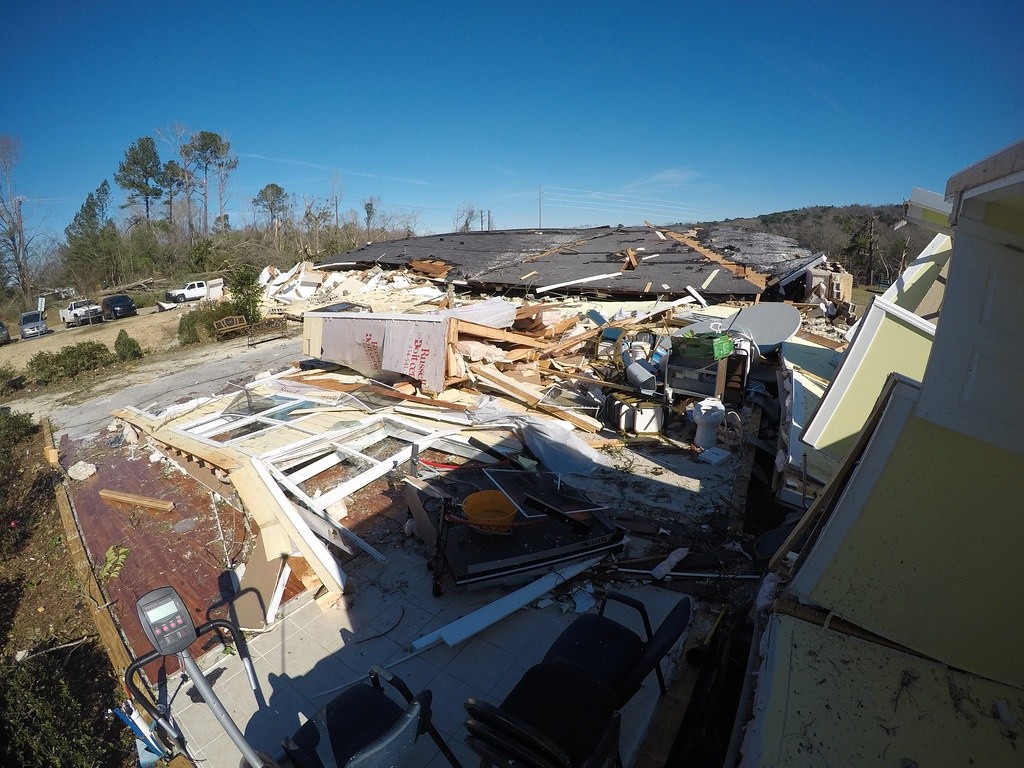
[462,590,691,768]
[586,309,629,360]
[604,393,684,446]
[280,666,462,768]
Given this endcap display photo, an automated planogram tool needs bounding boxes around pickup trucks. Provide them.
[58,299,103,329]
[166,281,207,304]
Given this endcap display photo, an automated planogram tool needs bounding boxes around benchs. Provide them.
[213,315,251,344]
[248,314,290,349]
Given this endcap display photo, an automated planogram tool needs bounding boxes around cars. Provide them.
[102,295,137,320]
[0,318,11,347]
[18,311,48,339]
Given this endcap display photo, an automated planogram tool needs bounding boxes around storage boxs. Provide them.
[671,332,734,360]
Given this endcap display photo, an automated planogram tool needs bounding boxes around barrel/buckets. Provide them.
[631,342,650,362]
[462,490,519,533]
